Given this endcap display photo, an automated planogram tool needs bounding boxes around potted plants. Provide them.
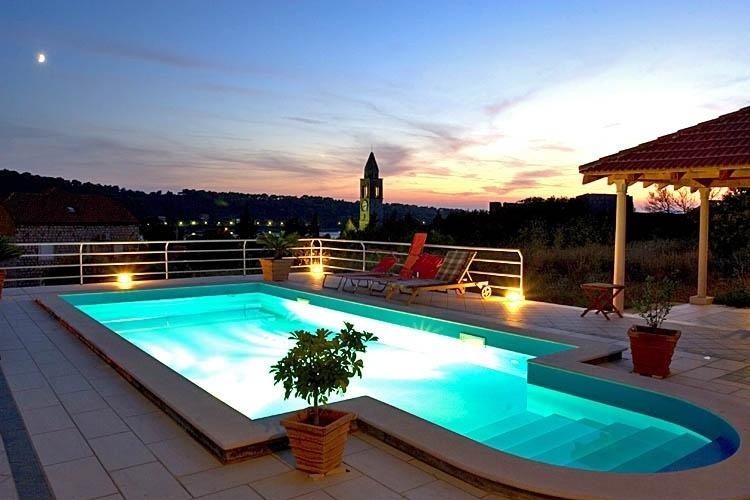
[256,229,300,281]
[0,233,26,300]
[269,321,378,474]
[628,269,682,377]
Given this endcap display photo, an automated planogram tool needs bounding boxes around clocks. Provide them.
[360,199,368,211]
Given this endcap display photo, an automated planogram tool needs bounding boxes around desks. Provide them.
[581,282,625,319]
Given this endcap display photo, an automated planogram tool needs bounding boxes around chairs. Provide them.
[322,249,492,305]
